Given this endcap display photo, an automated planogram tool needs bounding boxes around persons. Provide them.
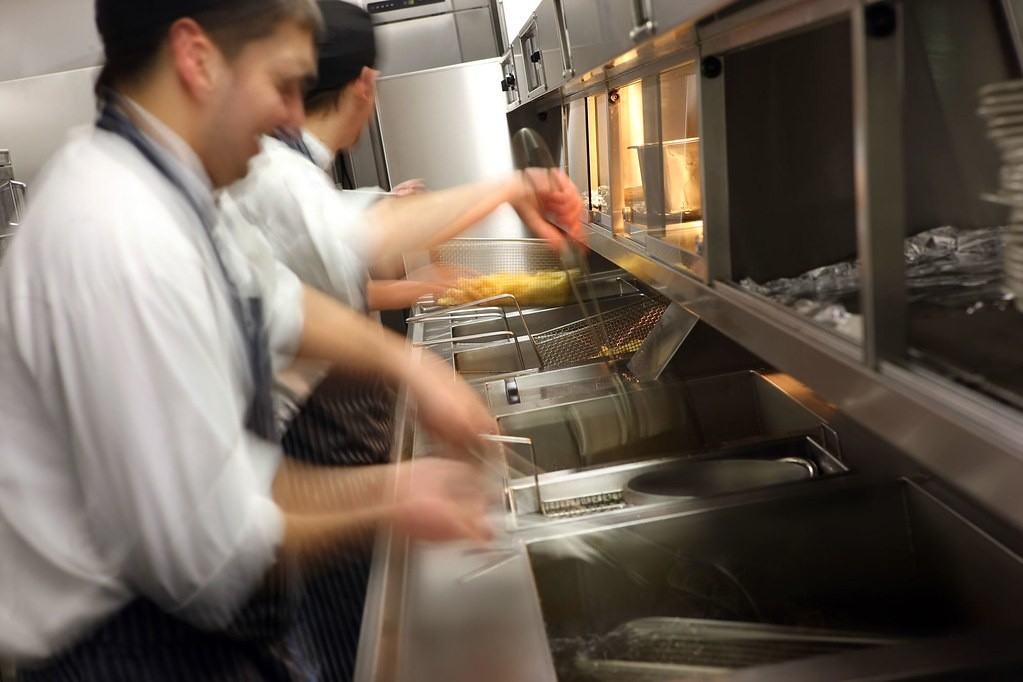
[0,0,501,682]
[226,0,587,682]
